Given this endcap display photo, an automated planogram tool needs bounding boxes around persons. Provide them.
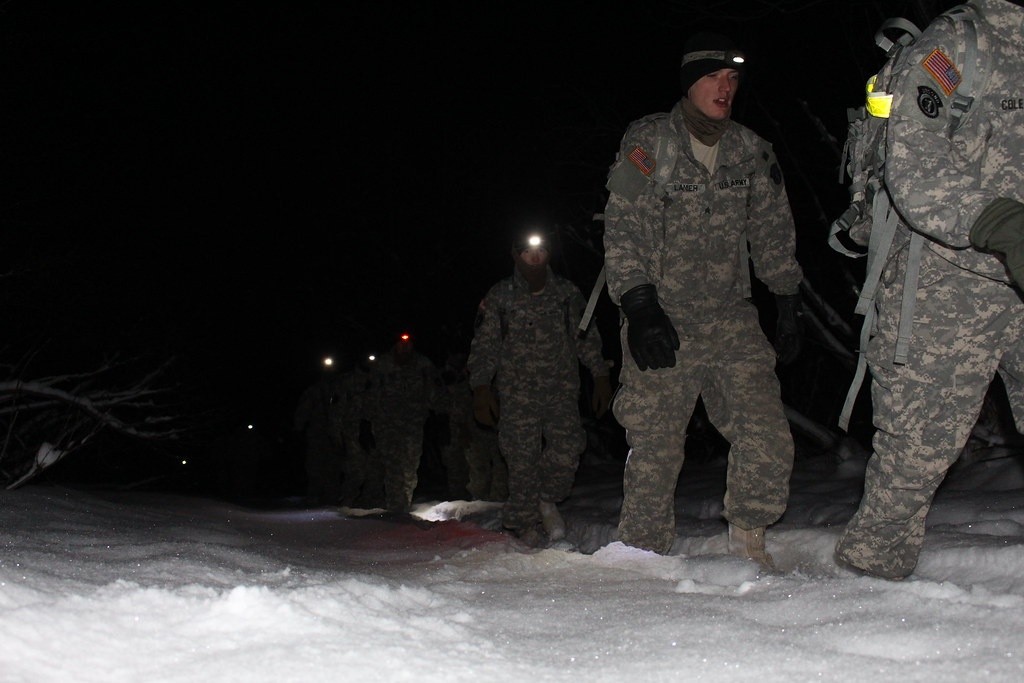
[466,229,614,545]
[834,0,1024,582]
[603,38,804,560]
[295,323,508,523]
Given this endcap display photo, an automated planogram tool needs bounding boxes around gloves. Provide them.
[472,384,500,426]
[620,284,680,371]
[593,376,613,418]
[774,292,805,365]
[969,197,1024,293]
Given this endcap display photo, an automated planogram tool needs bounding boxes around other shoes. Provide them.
[728,521,771,575]
[537,500,566,539]
[515,523,549,548]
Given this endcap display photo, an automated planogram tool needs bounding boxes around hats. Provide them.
[516,221,549,255]
[680,33,739,94]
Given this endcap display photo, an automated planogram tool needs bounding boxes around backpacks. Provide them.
[840,5,982,246]
